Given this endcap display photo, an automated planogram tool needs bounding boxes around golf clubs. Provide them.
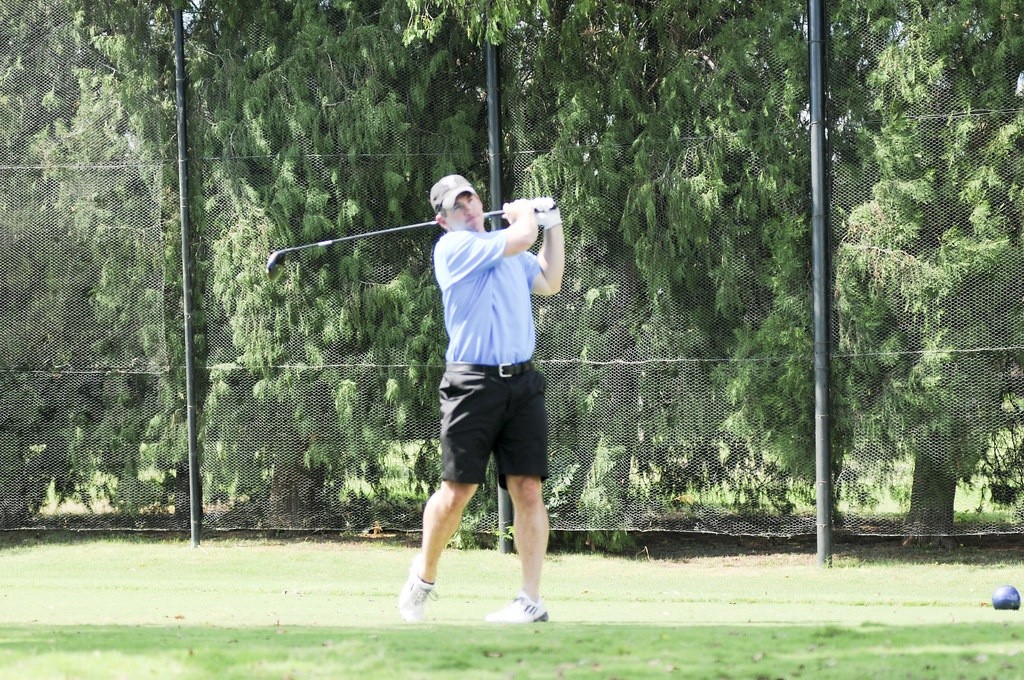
[266,200,559,283]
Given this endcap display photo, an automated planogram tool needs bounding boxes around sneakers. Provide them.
[511,592,548,623]
[399,559,437,620]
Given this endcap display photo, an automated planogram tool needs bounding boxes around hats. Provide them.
[429,174,476,215]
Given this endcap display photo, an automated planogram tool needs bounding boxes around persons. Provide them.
[401,175,565,622]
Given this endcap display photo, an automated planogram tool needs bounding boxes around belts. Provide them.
[446,359,532,378]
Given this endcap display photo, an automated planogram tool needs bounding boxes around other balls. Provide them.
[992,584,1021,610]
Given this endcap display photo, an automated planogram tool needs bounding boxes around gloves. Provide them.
[531,198,563,230]
